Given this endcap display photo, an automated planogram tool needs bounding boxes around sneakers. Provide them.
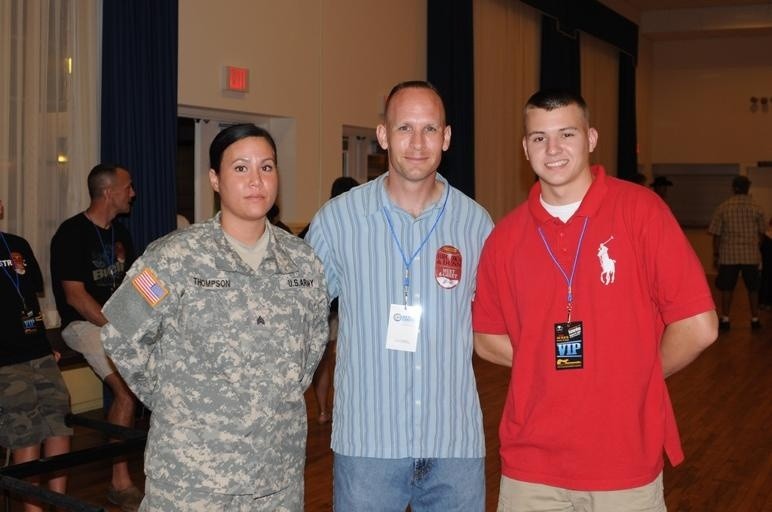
[751,321,762,329]
[719,318,730,329]
[107,483,143,512]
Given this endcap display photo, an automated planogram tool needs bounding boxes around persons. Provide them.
[101,121,328,512]
[0,204,78,512]
[51,164,143,512]
[757,218,772,315]
[472,84,716,512]
[627,174,646,186]
[707,175,762,332]
[299,175,360,424]
[647,174,674,199]
[304,80,496,512]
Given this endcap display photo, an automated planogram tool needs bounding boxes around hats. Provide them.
[649,177,673,187]
